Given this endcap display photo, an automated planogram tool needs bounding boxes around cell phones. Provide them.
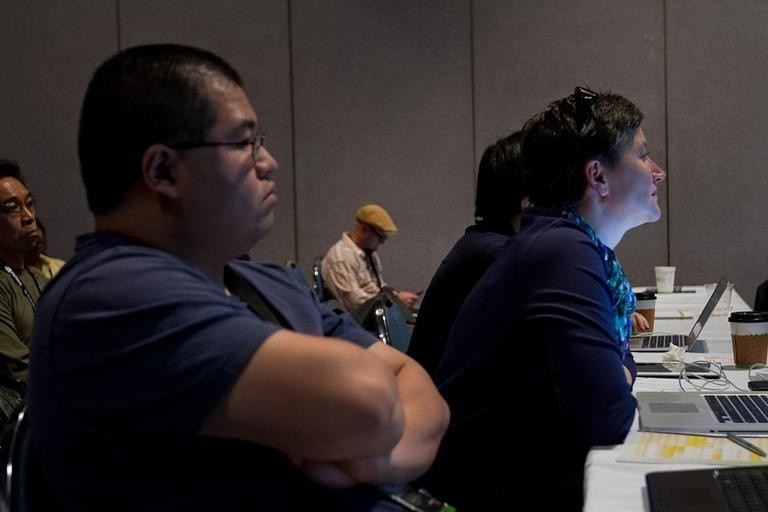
[416,289,424,295]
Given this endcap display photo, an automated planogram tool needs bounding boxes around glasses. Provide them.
[574,85,598,130]
[176,132,266,165]
[2,196,34,215]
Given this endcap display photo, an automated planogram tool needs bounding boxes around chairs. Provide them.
[312,253,327,301]
[370,288,410,360]
[285,258,309,290]
[0,394,31,512]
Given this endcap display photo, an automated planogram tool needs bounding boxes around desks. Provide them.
[574,271,767,510]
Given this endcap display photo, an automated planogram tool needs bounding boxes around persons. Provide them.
[24,218,67,281]
[0,156,52,475]
[418,88,668,512]
[21,44,452,512]
[403,128,652,369]
[321,205,419,324]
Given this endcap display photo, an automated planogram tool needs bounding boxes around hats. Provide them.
[355,204,398,235]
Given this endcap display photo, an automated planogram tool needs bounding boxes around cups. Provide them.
[728,310,766,369]
[654,266,677,294]
[635,292,657,335]
[705,282,733,317]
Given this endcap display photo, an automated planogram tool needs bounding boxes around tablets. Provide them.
[636,362,721,379]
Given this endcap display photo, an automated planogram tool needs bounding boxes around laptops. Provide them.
[629,276,729,352]
[646,465,768,512]
[636,390,768,438]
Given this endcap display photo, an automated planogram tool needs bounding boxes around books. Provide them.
[616,431,768,465]
